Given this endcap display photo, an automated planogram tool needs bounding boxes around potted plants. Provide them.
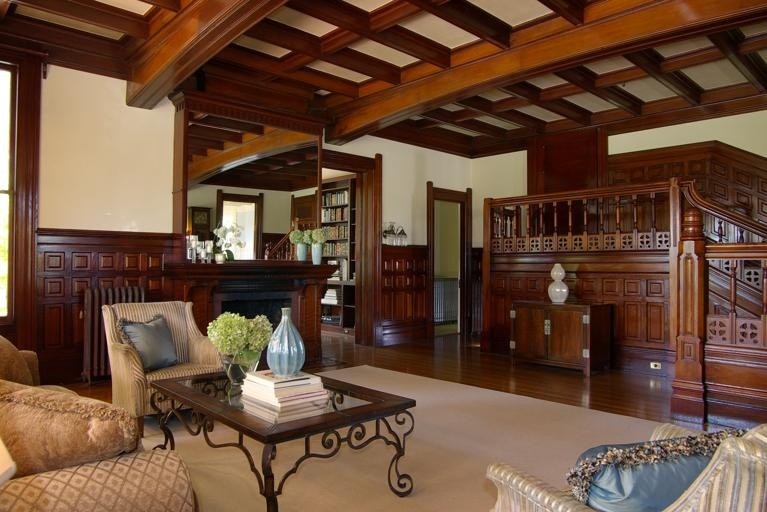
[290,231,306,260]
[207,310,273,386]
[306,229,326,265]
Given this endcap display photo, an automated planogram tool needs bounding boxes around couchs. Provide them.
[0,338,198,512]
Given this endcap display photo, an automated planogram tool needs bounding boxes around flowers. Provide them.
[212,223,246,253]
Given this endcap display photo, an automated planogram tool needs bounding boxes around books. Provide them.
[318,188,349,305]
[238,367,331,424]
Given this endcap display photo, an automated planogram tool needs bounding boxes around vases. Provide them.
[214,254,226,264]
[548,263,570,304]
[267,307,306,378]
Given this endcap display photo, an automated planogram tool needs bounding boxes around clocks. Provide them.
[188,206,212,239]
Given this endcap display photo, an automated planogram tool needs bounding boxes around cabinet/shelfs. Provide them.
[506,301,614,378]
[319,173,357,335]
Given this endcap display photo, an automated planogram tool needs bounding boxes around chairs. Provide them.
[99,300,226,437]
[488,423,767,512]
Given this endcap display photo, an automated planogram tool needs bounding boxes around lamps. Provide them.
[382,223,408,248]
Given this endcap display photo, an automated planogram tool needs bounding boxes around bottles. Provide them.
[186,235,212,264]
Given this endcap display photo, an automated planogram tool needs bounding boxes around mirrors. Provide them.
[185,108,319,262]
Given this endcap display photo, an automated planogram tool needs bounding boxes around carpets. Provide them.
[137,364,665,512]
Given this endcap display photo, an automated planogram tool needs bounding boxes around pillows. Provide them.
[116,314,177,369]
[0,378,138,478]
[567,428,749,512]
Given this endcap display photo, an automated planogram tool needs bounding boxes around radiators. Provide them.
[80,286,146,383]
